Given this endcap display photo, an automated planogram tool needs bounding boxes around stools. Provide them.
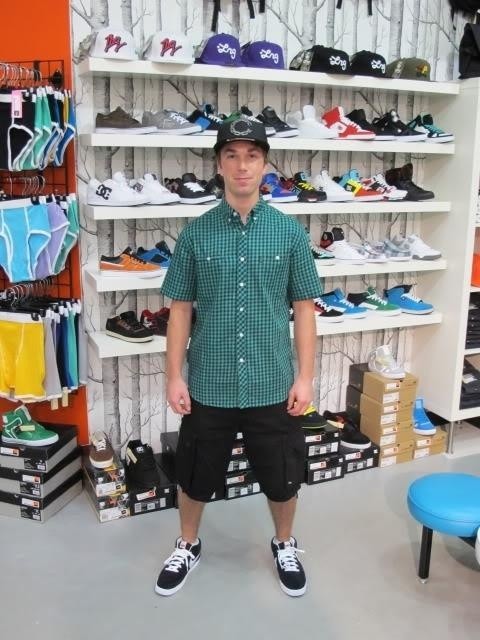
[405,470,480,583]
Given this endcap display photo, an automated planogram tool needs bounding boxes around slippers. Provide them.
[300,412,330,428]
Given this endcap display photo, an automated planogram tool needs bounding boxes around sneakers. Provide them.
[270,536,306,596]
[334,414,373,451]
[0,405,58,447]
[88,431,117,471]
[311,284,434,323]
[94,103,227,135]
[260,163,434,203]
[121,438,162,492]
[134,240,173,269]
[87,172,225,207]
[306,227,442,266]
[155,537,203,596]
[411,397,438,438]
[105,307,198,343]
[241,105,453,143]
[99,246,164,279]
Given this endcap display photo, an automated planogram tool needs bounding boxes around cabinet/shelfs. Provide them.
[410,72,480,454]
[82,51,461,362]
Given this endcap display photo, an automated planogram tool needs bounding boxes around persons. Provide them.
[157,115,316,596]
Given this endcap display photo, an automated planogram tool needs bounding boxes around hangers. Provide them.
[0,65,77,321]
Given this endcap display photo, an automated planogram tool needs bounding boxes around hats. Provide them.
[213,114,270,154]
[95,31,439,84]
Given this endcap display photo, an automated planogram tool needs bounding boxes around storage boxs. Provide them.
[344,364,450,468]
[78,412,382,525]
[1,420,84,524]
